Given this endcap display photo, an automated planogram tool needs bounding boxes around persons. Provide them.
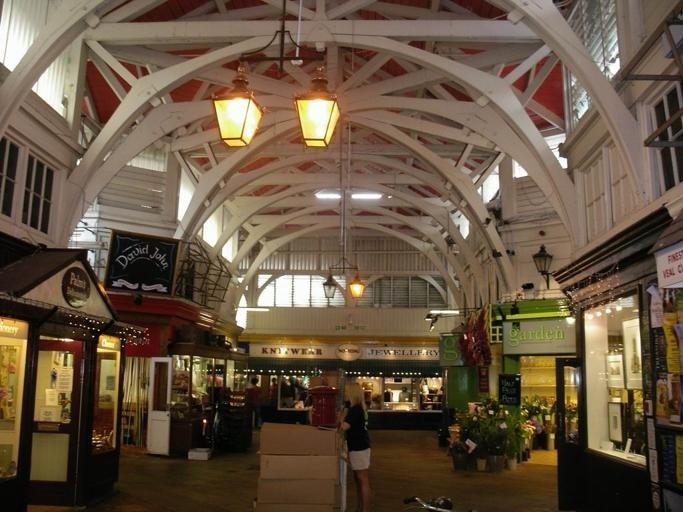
[340,381,372,512]
[205,373,313,431]
[549,395,567,449]
[358,380,444,409]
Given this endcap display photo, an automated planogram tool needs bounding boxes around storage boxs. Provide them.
[254,422,344,511]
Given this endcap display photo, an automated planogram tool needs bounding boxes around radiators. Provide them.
[454,399,536,460]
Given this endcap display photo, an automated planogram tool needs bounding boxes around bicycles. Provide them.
[403,494,474,512]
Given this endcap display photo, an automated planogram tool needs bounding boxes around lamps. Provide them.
[209,1,341,149]
[532,244,553,289]
[321,122,364,299]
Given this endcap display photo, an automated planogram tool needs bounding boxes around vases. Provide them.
[476,448,530,473]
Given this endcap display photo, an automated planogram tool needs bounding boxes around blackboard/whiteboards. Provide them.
[499,374,521,405]
[103,229,182,298]
